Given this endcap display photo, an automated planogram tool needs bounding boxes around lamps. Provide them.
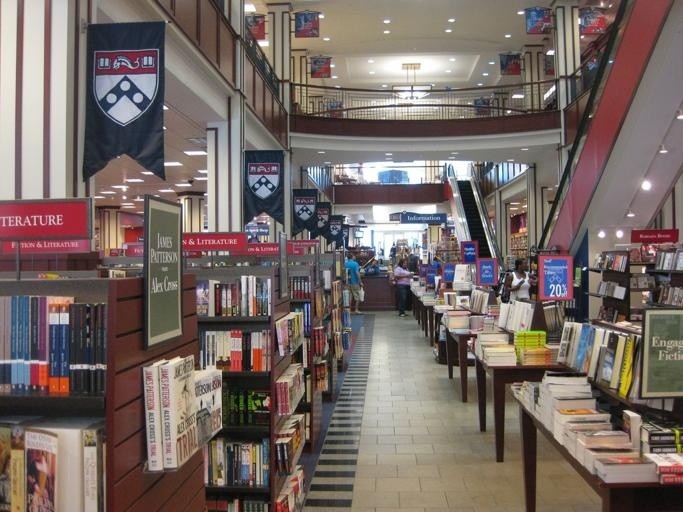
[625,209,635,219]
[659,144,667,155]
[676,110,683,123]
[391,63,433,100]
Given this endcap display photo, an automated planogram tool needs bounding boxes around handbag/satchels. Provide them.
[359,286,364,302]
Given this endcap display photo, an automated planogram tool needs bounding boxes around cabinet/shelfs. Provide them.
[505,319,683,512]
[0,267,210,511]
[443,287,534,403]
[102,253,351,511]
[580,247,650,324]
[409,277,473,360]
[508,233,529,268]
[349,240,415,311]
[468,298,573,462]
[645,248,683,310]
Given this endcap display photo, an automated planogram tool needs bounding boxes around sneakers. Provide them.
[399,313,405,317]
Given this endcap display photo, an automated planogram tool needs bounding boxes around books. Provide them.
[0,293,108,397]
[221,380,272,426]
[595,280,627,299]
[0,412,108,512]
[142,354,223,472]
[199,326,273,373]
[629,272,657,289]
[510,320,683,485]
[597,305,627,324]
[203,436,272,488]
[657,284,683,307]
[592,252,628,273]
[195,274,272,317]
[205,495,271,512]
[274,275,353,512]
[409,263,560,367]
[655,247,683,271]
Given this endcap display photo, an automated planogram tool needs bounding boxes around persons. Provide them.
[393,257,414,317]
[345,254,366,315]
[378,242,421,264]
[503,257,538,300]
